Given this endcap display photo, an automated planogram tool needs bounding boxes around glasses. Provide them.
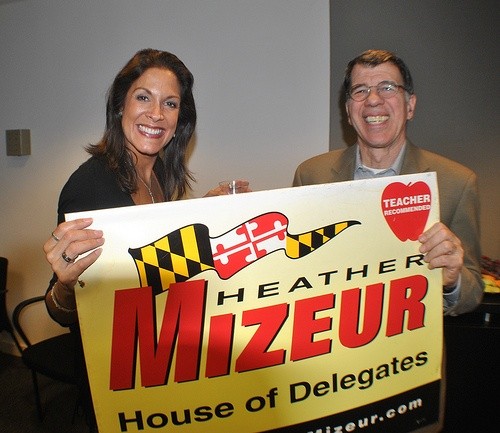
[347,80,410,102]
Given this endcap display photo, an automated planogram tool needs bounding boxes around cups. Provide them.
[218,180,250,195]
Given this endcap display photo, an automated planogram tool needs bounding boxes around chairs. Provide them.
[13,295,96,433]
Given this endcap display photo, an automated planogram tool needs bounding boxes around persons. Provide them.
[42,47,258,433]
[292,49,484,433]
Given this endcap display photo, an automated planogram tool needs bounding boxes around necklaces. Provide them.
[134,173,155,203]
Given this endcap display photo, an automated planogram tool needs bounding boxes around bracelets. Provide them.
[50,283,79,312]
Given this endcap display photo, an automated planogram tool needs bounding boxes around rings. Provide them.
[51,231,61,243]
[62,251,75,263]
